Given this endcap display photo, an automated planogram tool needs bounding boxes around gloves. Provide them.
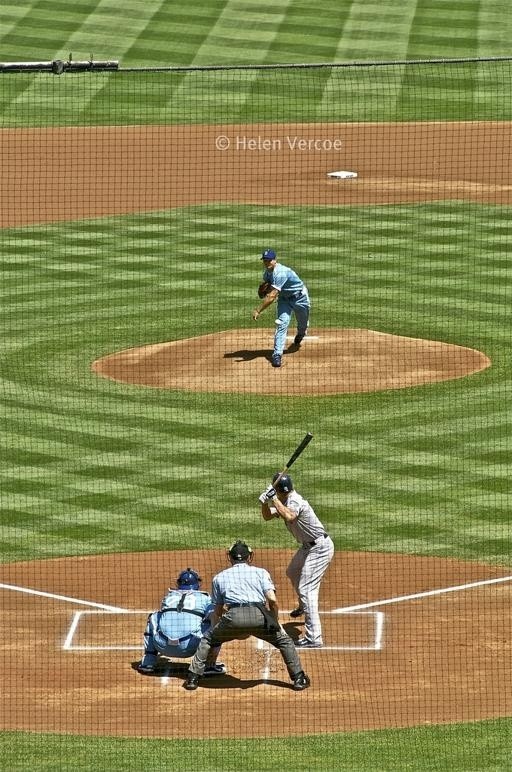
[263,485,278,501]
[258,492,270,505]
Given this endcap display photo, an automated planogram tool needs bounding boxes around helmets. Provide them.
[272,473,293,493]
[176,567,202,590]
[226,540,255,564]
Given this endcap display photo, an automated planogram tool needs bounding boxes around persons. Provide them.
[137,567,227,676]
[181,541,311,692]
[259,471,336,649]
[248,251,311,368]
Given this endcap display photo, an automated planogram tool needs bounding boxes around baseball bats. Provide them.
[273,431,314,491]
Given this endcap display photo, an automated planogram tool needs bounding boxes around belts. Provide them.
[157,628,192,642]
[228,603,264,608]
[302,533,328,550]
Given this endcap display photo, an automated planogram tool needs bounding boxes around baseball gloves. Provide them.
[259,281,272,296]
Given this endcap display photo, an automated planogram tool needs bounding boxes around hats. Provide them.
[262,250,277,259]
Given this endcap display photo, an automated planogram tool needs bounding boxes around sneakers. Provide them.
[271,354,282,367]
[290,609,305,617]
[137,664,155,672]
[293,636,323,647]
[186,673,199,689]
[294,675,310,691]
[203,664,225,677]
[294,343,301,351]
